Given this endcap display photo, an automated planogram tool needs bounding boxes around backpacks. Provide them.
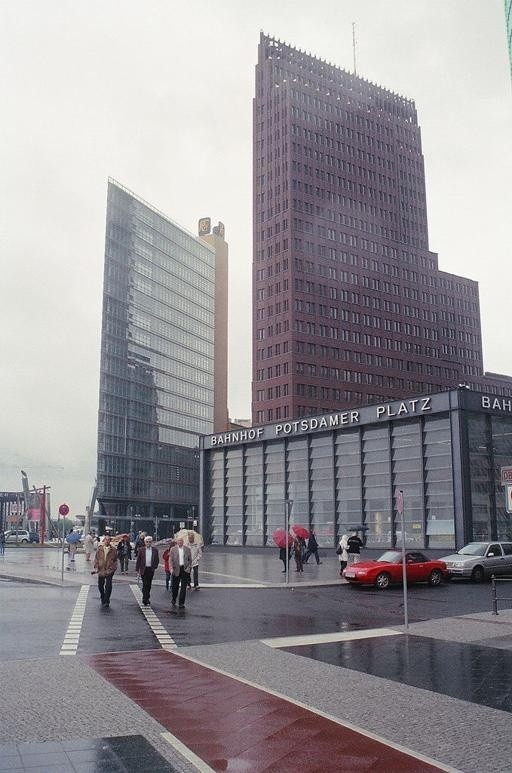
[336,546,342,555]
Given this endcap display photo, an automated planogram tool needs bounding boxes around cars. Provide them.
[436,539,512,579]
[29,532,40,543]
[92,534,135,550]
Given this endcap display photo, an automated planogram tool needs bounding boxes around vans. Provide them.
[5,529,31,544]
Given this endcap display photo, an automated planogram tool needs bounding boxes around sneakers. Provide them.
[172,600,176,605]
[179,604,185,609]
[103,602,110,608]
[143,599,150,605]
[195,586,200,590]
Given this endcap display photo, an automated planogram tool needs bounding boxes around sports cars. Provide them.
[340,547,450,589]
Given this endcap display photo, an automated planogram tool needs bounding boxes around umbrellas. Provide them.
[345,525,369,534]
[271,528,293,549]
[292,525,309,539]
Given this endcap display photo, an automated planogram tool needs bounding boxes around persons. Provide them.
[338,535,351,576]
[304,529,323,565]
[0,531,6,555]
[300,537,306,564]
[346,531,364,565]
[45,527,204,608]
[279,546,291,573]
[294,536,304,572]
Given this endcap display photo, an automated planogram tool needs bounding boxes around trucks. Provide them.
[387,531,416,544]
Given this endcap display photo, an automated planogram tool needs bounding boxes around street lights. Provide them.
[281,497,297,582]
